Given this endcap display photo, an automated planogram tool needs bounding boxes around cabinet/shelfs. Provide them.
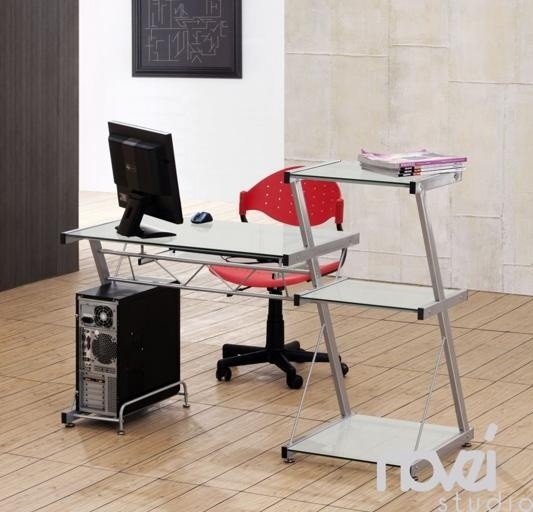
[279,157,478,482]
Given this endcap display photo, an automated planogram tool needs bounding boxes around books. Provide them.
[355,148,468,177]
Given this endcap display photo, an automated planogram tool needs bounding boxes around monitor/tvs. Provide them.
[107,120,182,238]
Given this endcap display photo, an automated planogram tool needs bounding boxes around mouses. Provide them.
[191,211,213,224]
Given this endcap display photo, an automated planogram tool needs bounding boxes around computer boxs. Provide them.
[76,277,180,418]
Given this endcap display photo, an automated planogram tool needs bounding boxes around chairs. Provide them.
[213,164,348,390]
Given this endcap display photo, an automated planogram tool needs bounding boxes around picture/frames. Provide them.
[130,0,243,78]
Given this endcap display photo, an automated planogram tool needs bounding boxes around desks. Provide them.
[56,210,359,400]
